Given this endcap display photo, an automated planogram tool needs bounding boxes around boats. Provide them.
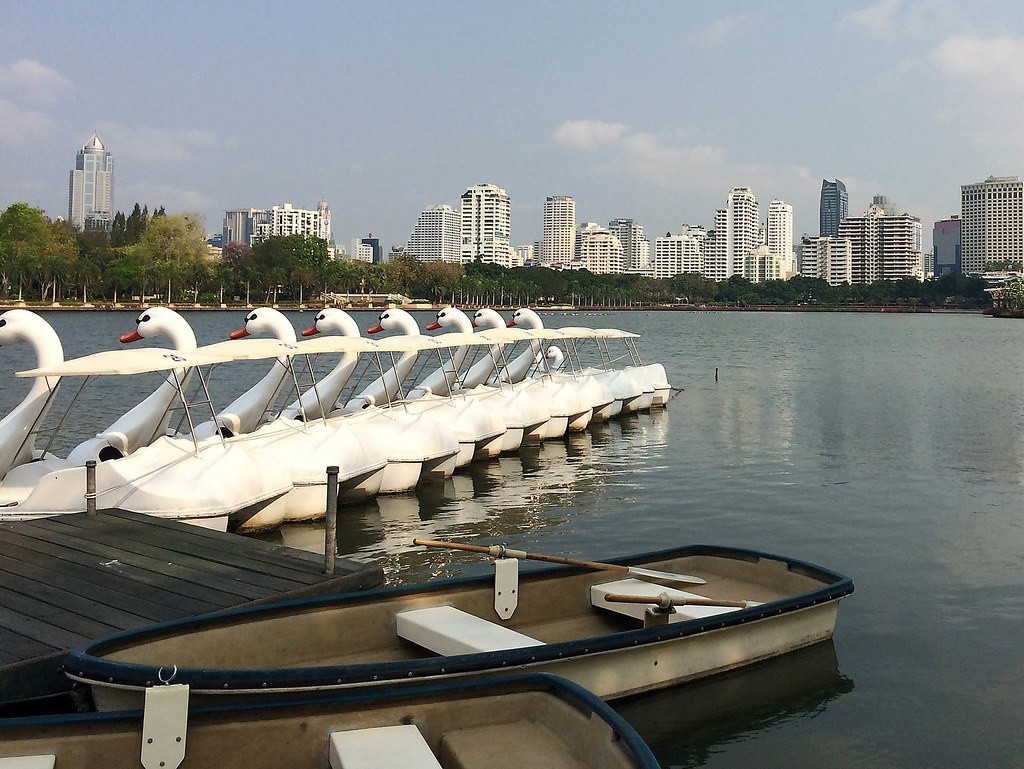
[0,305,671,535]
[62,532,858,708]
[0,659,660,769]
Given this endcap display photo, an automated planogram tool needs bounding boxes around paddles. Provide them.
[603,592,770,612]
[413,537,711,584]
